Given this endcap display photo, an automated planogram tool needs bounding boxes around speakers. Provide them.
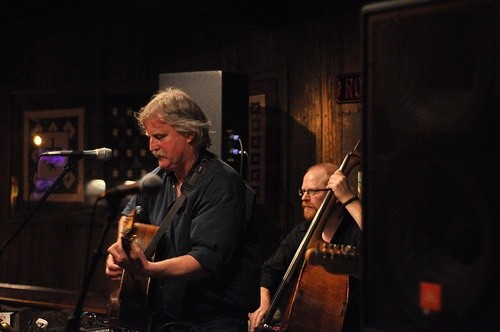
[159,69,248,186]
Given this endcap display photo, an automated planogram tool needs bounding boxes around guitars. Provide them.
[117,209,161,328]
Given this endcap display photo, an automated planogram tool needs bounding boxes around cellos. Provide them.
[254,137,362,332]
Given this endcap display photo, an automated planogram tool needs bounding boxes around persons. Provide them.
[104,86,256,332]
[248,162,362,332]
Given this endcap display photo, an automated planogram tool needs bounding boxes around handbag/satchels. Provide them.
[25,311,119,332]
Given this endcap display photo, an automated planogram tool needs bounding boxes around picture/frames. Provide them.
[22,107,87,202]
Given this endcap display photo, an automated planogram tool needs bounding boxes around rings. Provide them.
[105,276,112,281]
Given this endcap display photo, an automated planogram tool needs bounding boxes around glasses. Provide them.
[298,188,329,197]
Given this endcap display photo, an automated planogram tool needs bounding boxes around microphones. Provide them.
[104,174,163,200]
[42,147,112,161]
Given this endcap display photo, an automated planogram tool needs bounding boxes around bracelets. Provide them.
[342,196,359,208]
